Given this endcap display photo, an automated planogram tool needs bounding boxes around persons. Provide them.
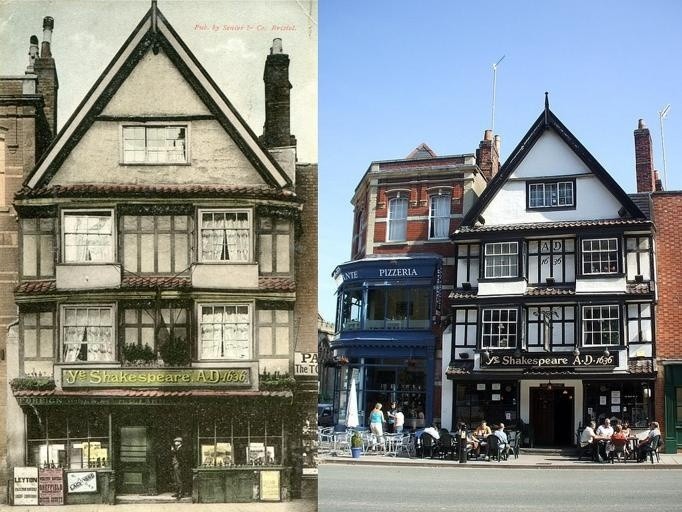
[369,402,387,452]
[418,420,511,463]
[387,401,398,433]
[387,406,405,433]
[578,418,661,463]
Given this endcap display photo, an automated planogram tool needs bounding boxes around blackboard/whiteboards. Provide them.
[13,467,97,506]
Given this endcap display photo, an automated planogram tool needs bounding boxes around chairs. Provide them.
[575,428,664,464]
[316,424,522,463]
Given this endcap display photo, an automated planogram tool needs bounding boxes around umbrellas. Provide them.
[345,379,359,428]
